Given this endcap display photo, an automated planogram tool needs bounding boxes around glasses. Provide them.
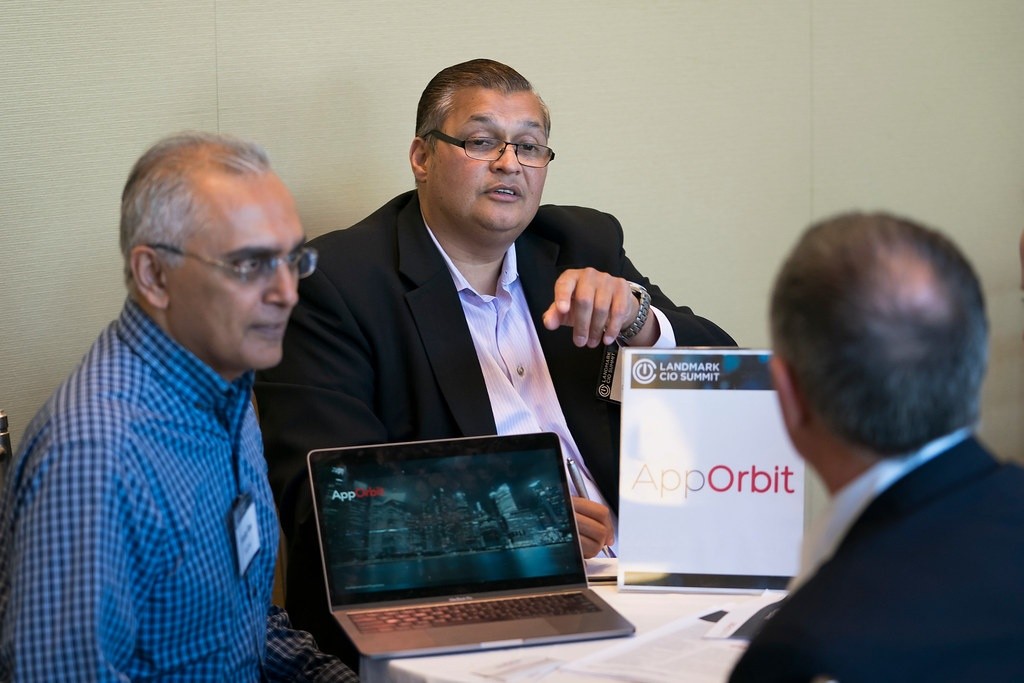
[429,129,556,168]
[151,241,319,280]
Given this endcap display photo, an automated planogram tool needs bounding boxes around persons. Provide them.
[726,211,1024,683]
[0,132,359,683]
[252,59,738,675]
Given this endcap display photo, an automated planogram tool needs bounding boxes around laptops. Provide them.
[305,432,636,657]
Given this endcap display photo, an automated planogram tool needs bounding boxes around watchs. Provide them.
[618,281,651,342]
